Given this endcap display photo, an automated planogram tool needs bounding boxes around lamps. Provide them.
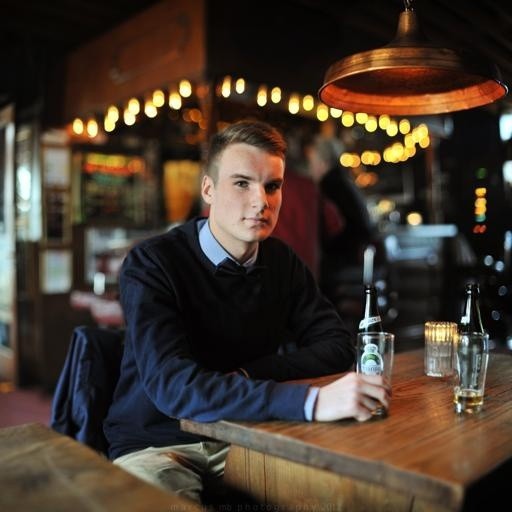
[318,0,510,117]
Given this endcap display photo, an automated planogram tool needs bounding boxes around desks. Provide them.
[179,341,512,512]
[0,421,207,512]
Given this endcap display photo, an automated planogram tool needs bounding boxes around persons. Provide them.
[305,134,388,334]
[200,134,344,283]
[100,119,394,511]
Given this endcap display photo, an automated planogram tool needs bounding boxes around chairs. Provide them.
[50,325,126,462]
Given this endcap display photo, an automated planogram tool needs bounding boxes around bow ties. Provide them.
[212,257,267,286]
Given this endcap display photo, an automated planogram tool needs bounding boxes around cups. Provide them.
[353,331,397,419]
[451,331,489,416]
[421,320,457,379]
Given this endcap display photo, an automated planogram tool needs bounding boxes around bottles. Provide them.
[356,281,387,380]
[456,279,487,389]
